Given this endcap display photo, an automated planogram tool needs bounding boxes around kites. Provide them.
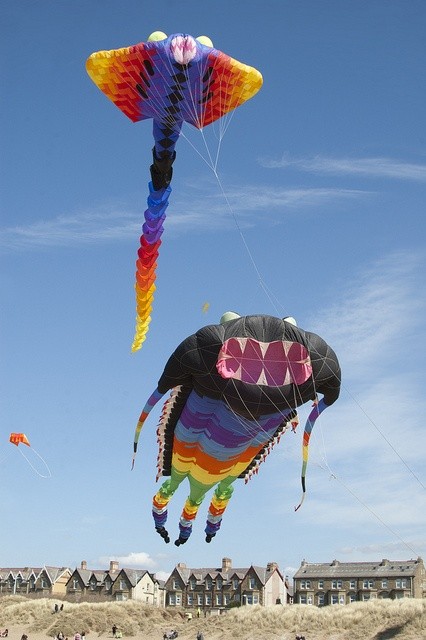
[133,311,341,546]
[85,30,263,352]
[9,433,52,479]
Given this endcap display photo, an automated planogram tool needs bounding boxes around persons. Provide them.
[75,630,86,640]
[112,624,119,639]
[196,631,204,640]
[163,629,178,640]
[56,632,68,640]
